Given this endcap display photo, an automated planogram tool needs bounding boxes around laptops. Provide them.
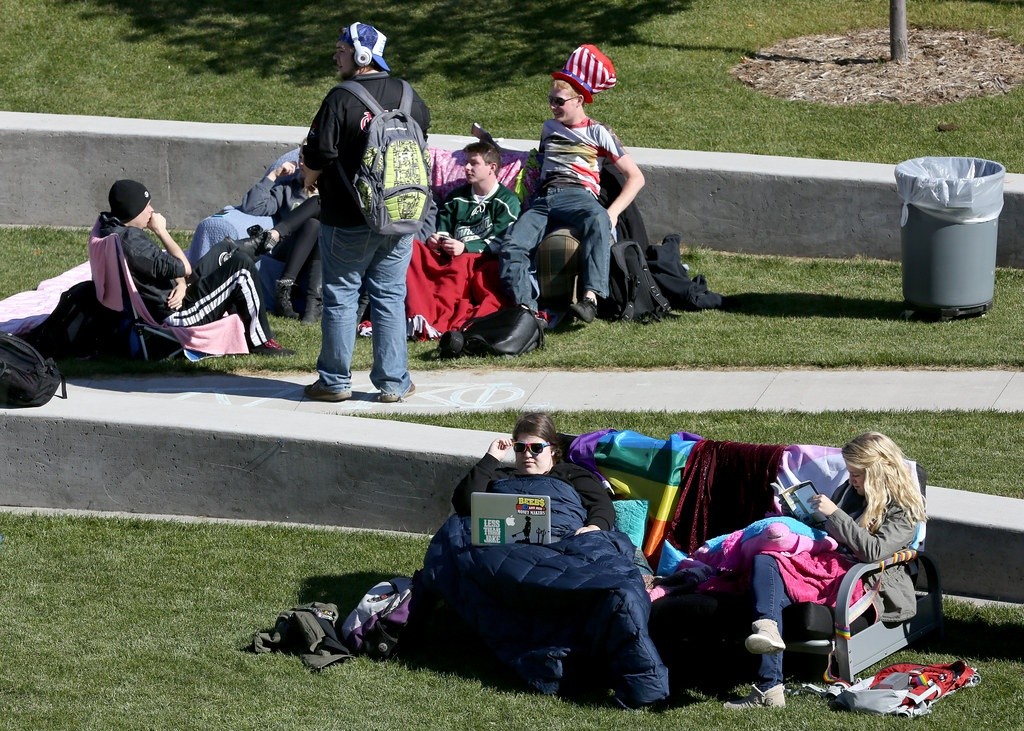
[471,493,551,547]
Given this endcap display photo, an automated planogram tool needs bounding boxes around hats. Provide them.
[108,179,151,223]
[551,44,617,103]
[338,24,391,72]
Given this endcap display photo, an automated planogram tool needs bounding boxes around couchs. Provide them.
[189,137,632,313]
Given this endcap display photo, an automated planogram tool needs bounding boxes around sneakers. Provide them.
[569,296,598,324]
[249,338,296,355]
[380,382,415,402]
[723,684,787,710]
[304,379,352,401]
[745,619,786,655]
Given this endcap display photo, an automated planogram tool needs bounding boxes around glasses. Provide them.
[548,95,579,106]
[512,441,551,455]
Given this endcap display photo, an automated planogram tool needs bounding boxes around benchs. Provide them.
[424,433,946,688]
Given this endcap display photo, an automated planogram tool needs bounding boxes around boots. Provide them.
[273,281,299,319]
[356,298,369,334]
[300,260,323,325]
[224,225,278,263]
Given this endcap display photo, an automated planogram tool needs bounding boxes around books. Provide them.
[770,480,828,528]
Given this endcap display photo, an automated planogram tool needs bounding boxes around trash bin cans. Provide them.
[894,156,1005,322]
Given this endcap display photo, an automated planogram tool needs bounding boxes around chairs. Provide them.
[88,217,235,376]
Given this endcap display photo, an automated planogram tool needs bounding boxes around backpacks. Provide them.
[322,78,434,236]
[460,304,549,358]
[0,330,67,407]
[342,569,424,662]
[610,239,671,324]
[831,662,982,718]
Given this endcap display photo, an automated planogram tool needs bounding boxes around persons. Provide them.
[98,180,281,351]
[451,411,614,537]
[302,22,430,403]
[414,141,520,258]
[242,137,370,332]
[499,69,645,324]
[725,432,929,711]
